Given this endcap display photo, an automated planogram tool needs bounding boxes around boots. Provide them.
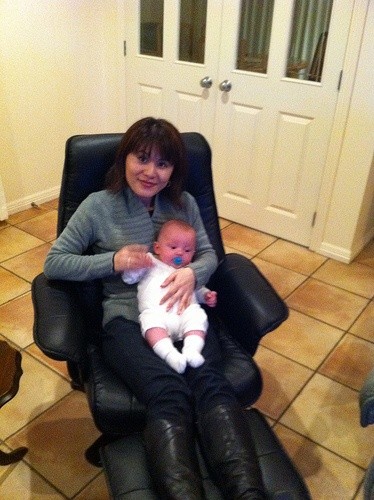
[144,419,207,500]
[195,403,268,500]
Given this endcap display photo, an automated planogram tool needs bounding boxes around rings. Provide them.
[125,255,133,269]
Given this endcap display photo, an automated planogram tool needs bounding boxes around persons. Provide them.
[120,216,218,374]
[42,116,275,500]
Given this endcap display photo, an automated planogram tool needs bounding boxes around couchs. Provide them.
[31,132,288,468]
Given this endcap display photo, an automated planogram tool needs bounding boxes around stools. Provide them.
[100,408,311,500]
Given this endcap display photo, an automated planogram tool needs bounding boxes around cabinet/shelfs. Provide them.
[124,0,374,264]
